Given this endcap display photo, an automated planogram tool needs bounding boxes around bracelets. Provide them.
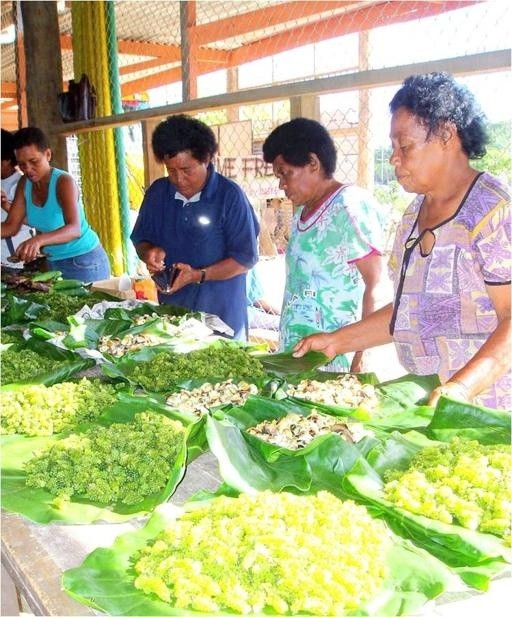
[197,267,205,284]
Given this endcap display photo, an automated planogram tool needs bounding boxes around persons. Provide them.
[1,129,36,272]
[291,71,512,414]
[1,127,109,284]
[263,117,381,372]
[130,115,260,344]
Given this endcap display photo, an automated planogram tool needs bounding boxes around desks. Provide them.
[0,265,512,615]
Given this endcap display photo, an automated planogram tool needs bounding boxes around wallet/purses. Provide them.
[151,266,176,291]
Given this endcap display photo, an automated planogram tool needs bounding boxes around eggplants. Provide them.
[6,277,50,293]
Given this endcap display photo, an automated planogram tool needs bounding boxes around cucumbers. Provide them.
[31,270,64,282]
[55,280,93,299]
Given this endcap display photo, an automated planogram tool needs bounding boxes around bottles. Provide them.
[134,275,159,303]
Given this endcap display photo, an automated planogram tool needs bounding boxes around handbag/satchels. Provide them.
[0,224,37,269]
[58,73,97,123]
